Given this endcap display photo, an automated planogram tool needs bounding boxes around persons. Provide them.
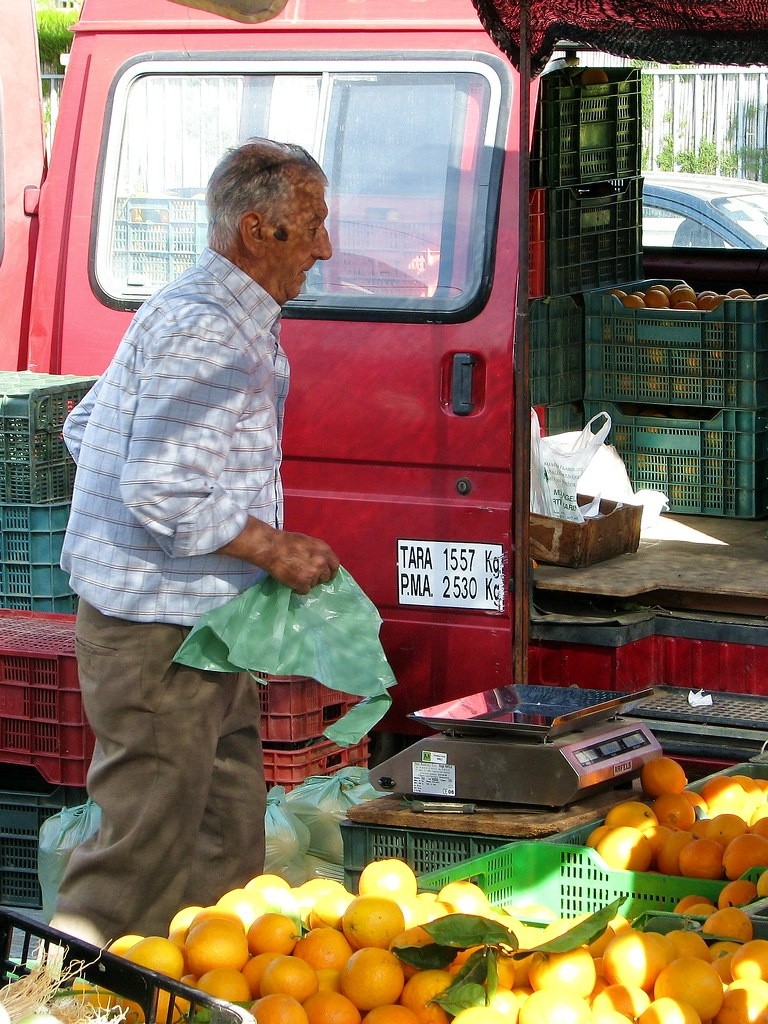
[672,216,726,248]
[48,137,340,951]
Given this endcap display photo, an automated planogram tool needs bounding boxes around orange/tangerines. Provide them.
[66,756,768,1024]
[590,283,768,507]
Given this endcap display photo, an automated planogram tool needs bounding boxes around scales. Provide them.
[366,684,660,810]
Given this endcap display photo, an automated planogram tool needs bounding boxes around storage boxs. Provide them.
[0,65,768,1024]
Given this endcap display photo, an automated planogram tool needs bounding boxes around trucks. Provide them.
[1,1,768,789]
